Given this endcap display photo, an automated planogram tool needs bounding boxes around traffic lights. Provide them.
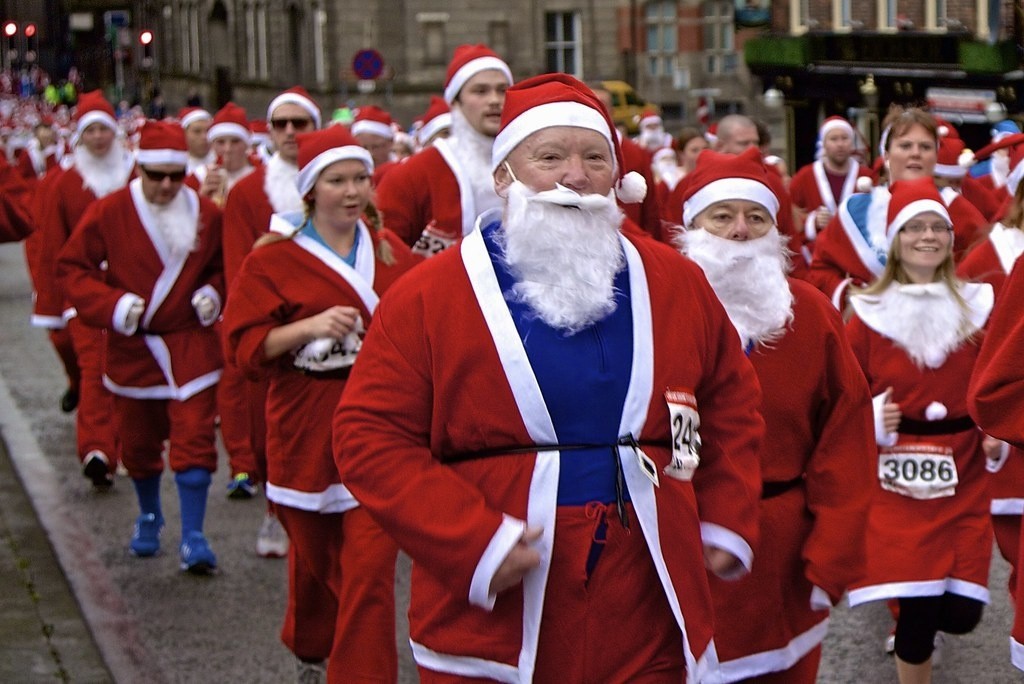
[137,30,157,71]
[3,20,20,63]
[21,20,38,66]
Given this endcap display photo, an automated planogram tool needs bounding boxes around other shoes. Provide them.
[932,632,942,666]
[61,390,79,412]
[296,662,327,684]
[226,473,257,499]
[885,635,895,653]
[82,451,114,488]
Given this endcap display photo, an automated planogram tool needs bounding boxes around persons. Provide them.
[0,44,1024,684]
[332,71,766,684]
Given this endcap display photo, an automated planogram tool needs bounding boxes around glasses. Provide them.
[141,165,186,183]
[900,221,954,237]
[268,118,315,131]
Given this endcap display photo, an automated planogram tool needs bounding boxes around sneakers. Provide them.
[177,530,217,572]
[129,513,165,555]
[258,515,289,558]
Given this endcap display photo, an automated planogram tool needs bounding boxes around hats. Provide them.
[885,177,955,256]
[178,102,274,154]
[819,116,854,146]
[77,89,118,138]
[267,85,321,131]
[957,134,1024,197]
[443,41,514,104]
[135,119,188,165]
[931,114,967,177]
[296,120,375,199]
[351,95,452,145]
[632,111,675,163]
[682,146,781,226]
[492,73,647,204]
